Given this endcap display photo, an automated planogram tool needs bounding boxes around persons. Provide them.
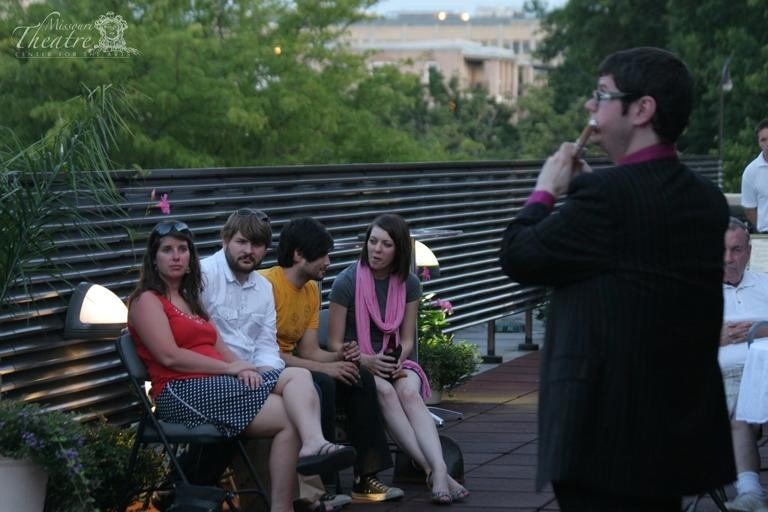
[326,214,471,504]
[718,218,768,512]
[128,219,362,511]
[255,216,405,506]
[197,207,354,507]
[498,46,739,512]
[740,119,768,232]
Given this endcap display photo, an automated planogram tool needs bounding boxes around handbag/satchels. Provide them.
[390,433,466,488]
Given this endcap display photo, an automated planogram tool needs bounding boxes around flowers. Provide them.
[0,397,165,512]
[416,268,482,393]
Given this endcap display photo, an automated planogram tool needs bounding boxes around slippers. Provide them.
[293,440,359,477]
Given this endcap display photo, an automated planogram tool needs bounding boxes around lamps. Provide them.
[409,239,440,268]
[61,280,131,341]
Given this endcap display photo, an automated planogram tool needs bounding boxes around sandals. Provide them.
[424,474,472,502]
[428,471,454,506]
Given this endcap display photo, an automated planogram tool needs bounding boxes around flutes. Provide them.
[572,120,596,159]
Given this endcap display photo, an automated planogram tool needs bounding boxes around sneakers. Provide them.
[351,475,406,503]
[723,488,768,512]
[308,491,353,508]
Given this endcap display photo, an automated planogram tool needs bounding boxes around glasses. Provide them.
[589,90,637,104]
[153,220,191,236]
[235,207,272,224]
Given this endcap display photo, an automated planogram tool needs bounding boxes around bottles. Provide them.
[383,332,397,380]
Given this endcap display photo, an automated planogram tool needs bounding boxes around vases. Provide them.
[421,376,442,406]
[0,450,50,512]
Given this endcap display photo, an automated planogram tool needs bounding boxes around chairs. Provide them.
[316,309,407,491]
[115,331,271,512]
